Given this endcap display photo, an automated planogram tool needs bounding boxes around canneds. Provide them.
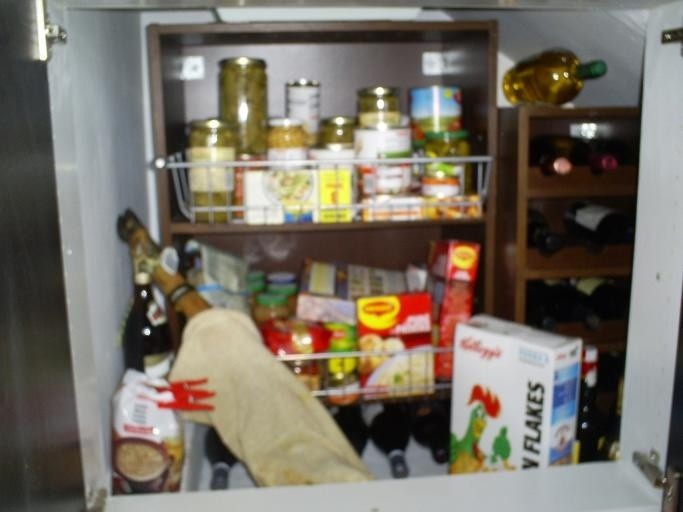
[183,57,470,224]
[245,269,298,324]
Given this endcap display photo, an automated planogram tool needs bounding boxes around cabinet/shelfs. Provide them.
[502,105,640,383]
[142,21,503,400]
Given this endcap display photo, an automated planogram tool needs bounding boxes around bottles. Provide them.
[574,346,627,463]
[119,269,177,381]
[192,395,450,494]
[501,42,609,107]
[525,128,634,335]
